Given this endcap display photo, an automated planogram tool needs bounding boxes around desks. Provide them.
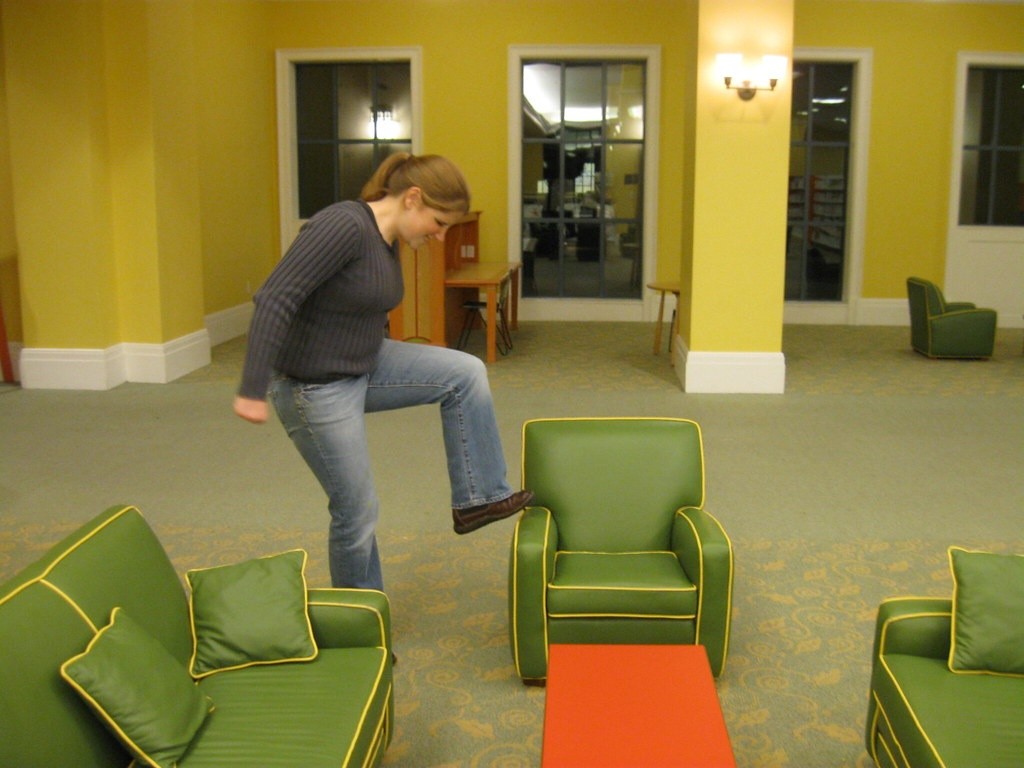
[523,238,537,296]
[647,278,681,355]
[541,644,737,768]
[445,262,523,365]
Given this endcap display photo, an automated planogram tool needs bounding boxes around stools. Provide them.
[455,302,514,356]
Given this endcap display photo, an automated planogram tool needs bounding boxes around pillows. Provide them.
[184,549,319,679]
[60,606,215,768]
[946,544,1024,678]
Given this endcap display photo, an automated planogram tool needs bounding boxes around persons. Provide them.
[231,151,535,666]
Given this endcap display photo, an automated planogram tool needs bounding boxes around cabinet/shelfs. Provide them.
[790,173,844,250]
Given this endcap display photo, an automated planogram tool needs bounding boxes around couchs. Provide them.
[618,223,637,258]
[863,597,1024,768]
[506,417,734,686]
[906,276,997,359]
[0,505,395,768]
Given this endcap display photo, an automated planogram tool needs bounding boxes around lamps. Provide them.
[715,51,790,100]
[369,83,394,120]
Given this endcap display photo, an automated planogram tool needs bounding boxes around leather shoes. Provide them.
[452,490,534,535]
